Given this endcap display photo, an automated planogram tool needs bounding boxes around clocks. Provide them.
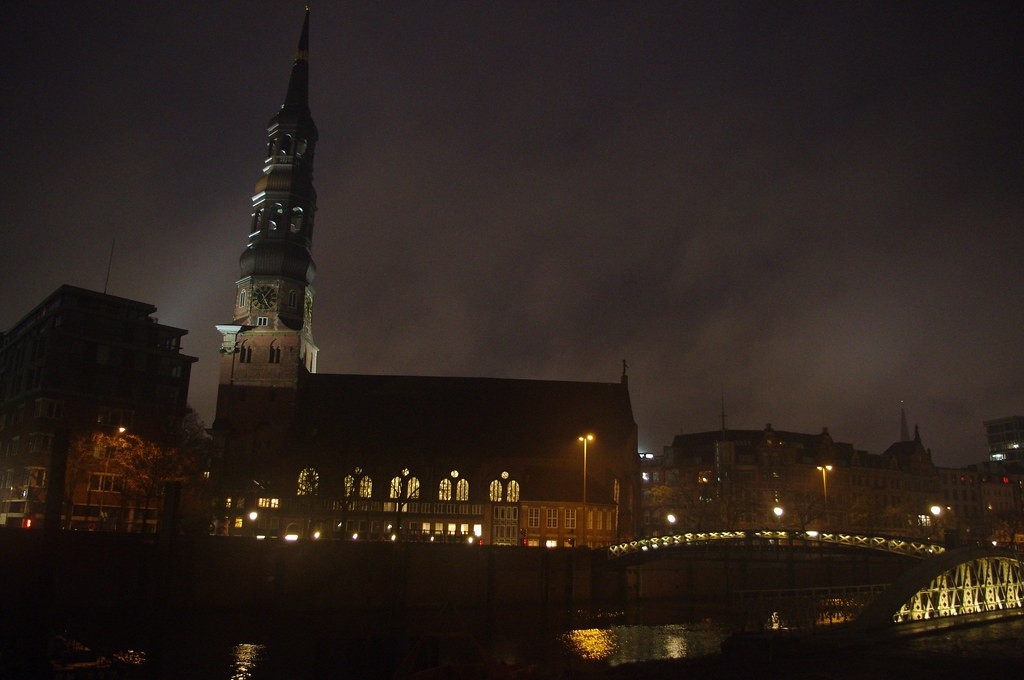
[251,285,278,312]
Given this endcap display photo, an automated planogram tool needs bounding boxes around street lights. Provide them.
[95,425,126,531]
[577,432,598,544]
[816,465,833,535]
